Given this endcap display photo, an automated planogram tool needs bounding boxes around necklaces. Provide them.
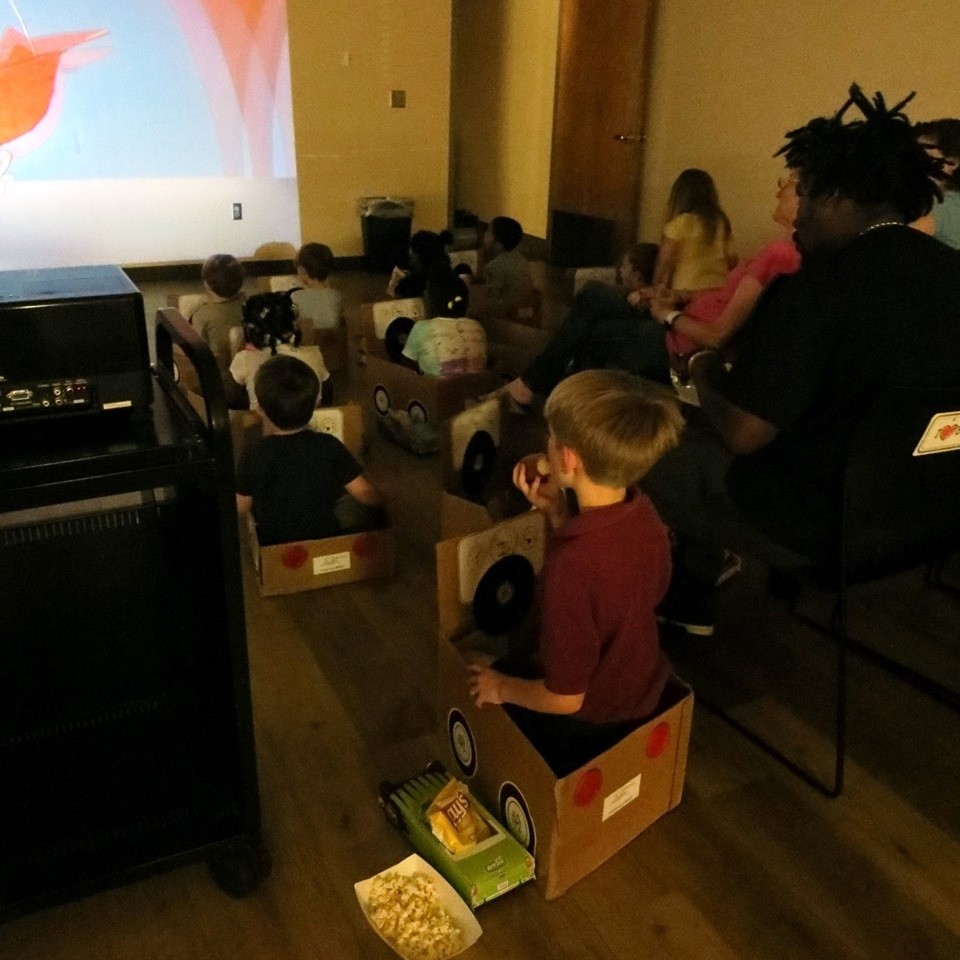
[854,221,909,242]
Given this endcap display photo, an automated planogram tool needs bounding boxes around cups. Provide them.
[656,290,674,305]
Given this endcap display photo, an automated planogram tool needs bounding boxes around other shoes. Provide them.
[465,392,534,418]
[654,601,714,635]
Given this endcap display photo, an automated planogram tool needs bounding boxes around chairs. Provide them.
[694,385,960,799]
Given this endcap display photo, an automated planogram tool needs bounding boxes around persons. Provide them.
[468,168,803,405]
[459,367,686,777]
[642,83,959,667]
[652,168,733,305]
[182,217,657,548]
[909,120,959,251]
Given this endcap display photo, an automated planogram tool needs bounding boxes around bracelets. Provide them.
[661,311,683,331]
[682,347,720,375]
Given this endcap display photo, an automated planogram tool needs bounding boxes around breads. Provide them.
[520,454,553,481]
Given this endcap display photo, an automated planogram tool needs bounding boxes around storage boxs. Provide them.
[178,249,695,900]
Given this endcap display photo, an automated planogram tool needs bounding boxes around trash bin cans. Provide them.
[359,195,415,274]
